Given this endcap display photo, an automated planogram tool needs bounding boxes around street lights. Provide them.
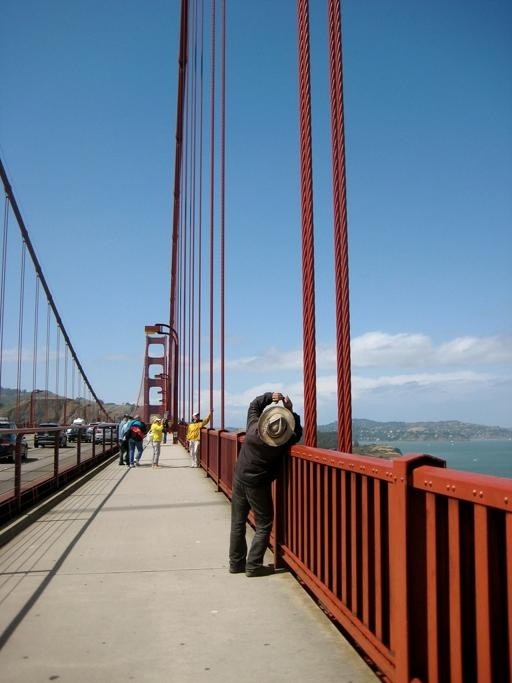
[144,321,179,444]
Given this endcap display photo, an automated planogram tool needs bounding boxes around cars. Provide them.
[66,417,119,444]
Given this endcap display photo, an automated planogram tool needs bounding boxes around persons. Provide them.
[229,390,302,576]
[121,415,143,466]
[127,418,146,467]
[179,418,186,425]
[185,409,213,468]
[118,414,129,464]
[162,419,170,443]
[149,417,165,468]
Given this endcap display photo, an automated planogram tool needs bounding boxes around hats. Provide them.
[256,407,297,448]
[154,417,161,422]
[135,415,140,419]
[193,412,200,416]
[123,413,130,417]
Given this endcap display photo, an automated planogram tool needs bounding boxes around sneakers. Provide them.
[119,459,162,469]
[229,566,246,573]
[246,568,269,576]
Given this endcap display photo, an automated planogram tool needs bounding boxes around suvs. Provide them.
[34,422,68,448]
[0,421,29,460]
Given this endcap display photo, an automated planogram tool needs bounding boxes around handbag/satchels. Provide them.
[147,429,153,442]
